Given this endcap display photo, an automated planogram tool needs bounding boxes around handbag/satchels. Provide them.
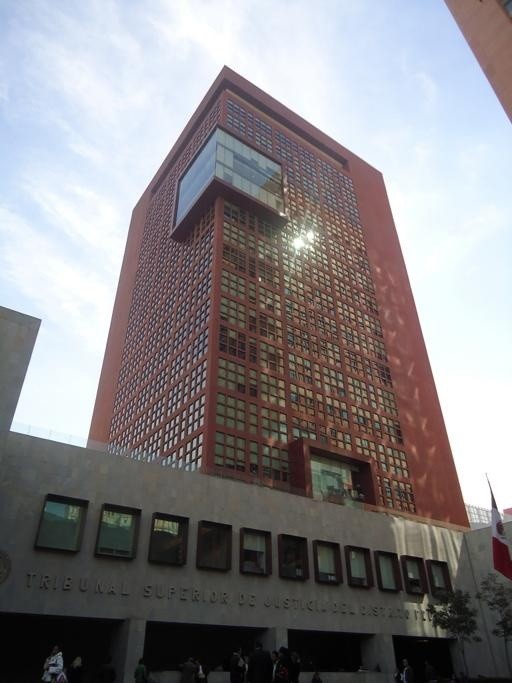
[56,671,68,683]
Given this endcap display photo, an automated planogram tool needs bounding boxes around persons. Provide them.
[42,644,116,682]
[193,659,211,683]
[395,658,435,683]
[134,657,148,682]
[230,642,302,681]
[179,657,196,682]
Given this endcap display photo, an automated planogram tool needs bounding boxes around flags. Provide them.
[493,495,512,581]
